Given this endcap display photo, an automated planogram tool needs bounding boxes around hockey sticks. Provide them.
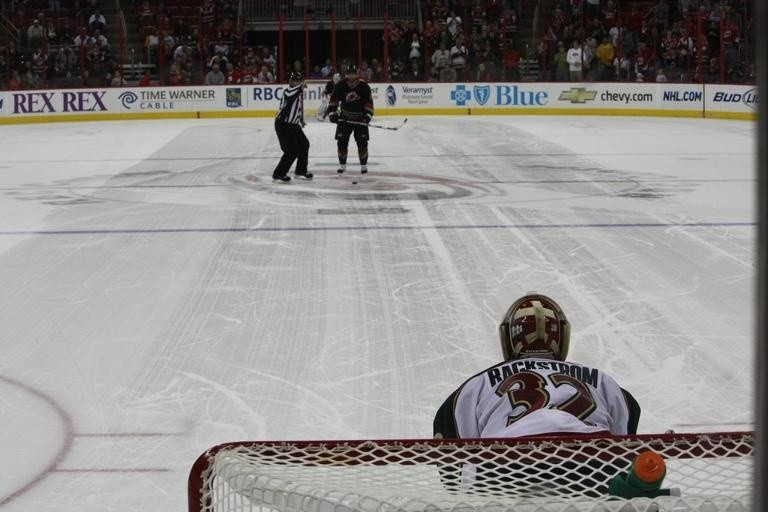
[339,118,407,130]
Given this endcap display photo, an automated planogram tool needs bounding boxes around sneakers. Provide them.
[295,172,313,178]
[337,165,346,173]
[273,174,290,182]
[361,165,367,174]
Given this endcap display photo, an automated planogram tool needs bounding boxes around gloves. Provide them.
[329,111,339,123]
[363,111,373,124]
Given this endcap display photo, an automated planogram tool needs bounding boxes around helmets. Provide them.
[333,73,342,84]
[500,296,570,361]
[345,65,360,82]
[289,72,304,88]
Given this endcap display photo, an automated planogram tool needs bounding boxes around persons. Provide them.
[429,293,641,500]
[328,65,373,174]
[316,74,346,121]
[271,71,314,182]
[0,0,277,89]
[387,0,758,86]
[290,52,388,82]
